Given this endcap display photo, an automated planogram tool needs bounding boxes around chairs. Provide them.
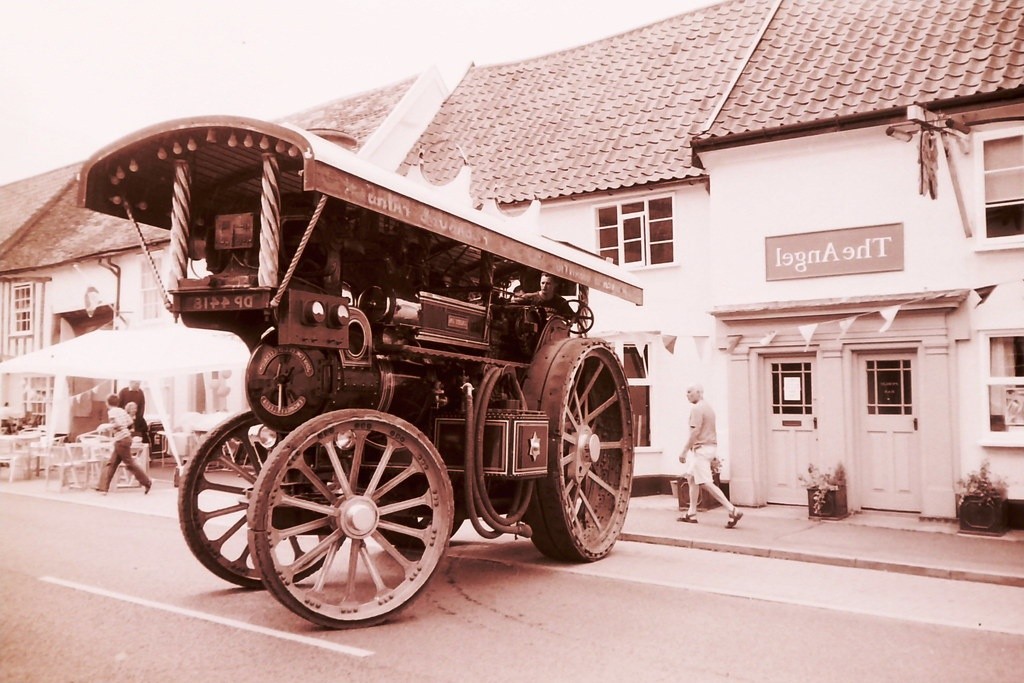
[0,427,149,488]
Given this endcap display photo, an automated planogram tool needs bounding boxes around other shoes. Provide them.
[144,480,152,494]
[94,489,108,496]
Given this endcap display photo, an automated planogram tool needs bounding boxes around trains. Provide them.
[79,111,640,629]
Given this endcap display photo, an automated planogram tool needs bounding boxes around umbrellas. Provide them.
[0,316,253,378]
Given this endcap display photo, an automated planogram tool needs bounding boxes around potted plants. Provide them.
[955,460,1009,537]
[677,458,724,512]
[797,460,849,521]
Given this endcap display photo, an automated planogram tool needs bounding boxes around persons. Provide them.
[119,380,145,417]
[677,385,743,529]
[125,401,151,444]
[513,286,528,302]
[522,273,574,316]
[93,395,152,495]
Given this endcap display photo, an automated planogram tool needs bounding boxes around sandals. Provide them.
[725,507,743,529]
[677,514,698,523]
